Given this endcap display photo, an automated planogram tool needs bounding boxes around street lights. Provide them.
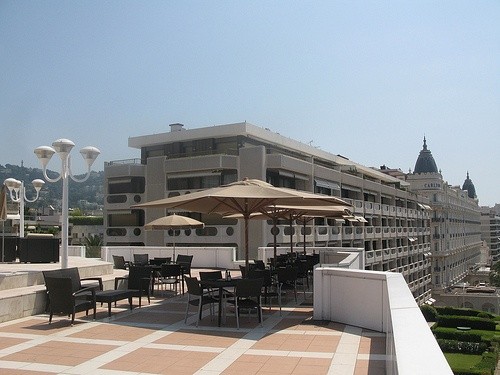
[33,139,102,269]
[4,177,45,237]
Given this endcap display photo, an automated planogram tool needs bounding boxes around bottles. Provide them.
[225,269,228,280]
[227,272,231,281]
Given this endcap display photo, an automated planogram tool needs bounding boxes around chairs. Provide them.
[42,249,321,331]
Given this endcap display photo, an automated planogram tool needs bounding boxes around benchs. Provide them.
[0,235,59,264]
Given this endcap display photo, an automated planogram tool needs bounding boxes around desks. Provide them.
[200,278,244,328]
[144,264,163,296]
[86,289,133,318]
